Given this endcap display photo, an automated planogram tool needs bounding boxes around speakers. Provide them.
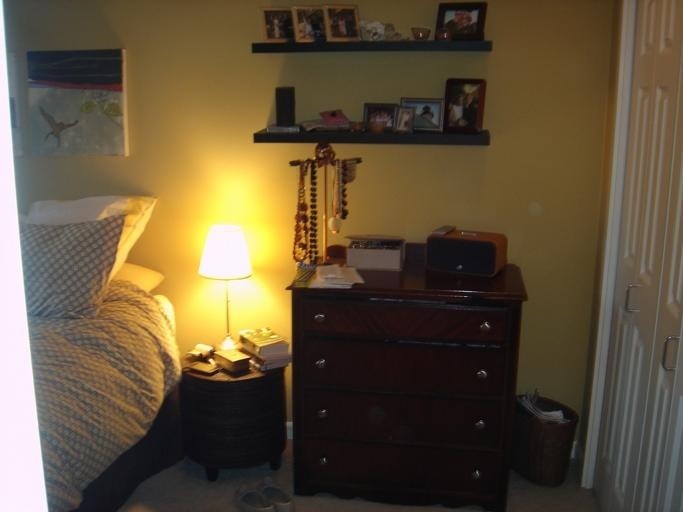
[427,230,507,278]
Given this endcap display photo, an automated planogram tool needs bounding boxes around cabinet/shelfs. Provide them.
[579,1,682,512]
[248,39,495,149]
[284,258,530,511]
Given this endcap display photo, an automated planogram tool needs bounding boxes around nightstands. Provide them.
[180,356,287,483]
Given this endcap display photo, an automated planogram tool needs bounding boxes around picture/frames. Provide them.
[391,105,416,135]
[255,7,293,45]
[399,96,444,133]
[363,103,397,134]
[322,4,362,43]
[290,5,326,44]
[434,1,489,40]
[443,78,487,133]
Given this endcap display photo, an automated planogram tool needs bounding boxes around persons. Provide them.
[463,91,477,127]
[400,109,411,130]
[446,94,465,126]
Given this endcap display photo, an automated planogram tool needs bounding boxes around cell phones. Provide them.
[190,363,219,375]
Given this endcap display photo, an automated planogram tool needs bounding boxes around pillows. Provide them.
[109,259,167,291]
[17,211,128,323]
[25,191,162,283]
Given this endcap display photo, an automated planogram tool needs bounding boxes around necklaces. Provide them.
[290,158,308,263]
[333,161,348,222]
[308,163,317,266]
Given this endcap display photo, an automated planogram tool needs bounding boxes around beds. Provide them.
[23,292,179,511]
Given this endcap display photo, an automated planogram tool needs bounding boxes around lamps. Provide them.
[195,223,256,351]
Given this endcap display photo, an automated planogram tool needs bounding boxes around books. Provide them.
[299,108,350,133]
[311,263,365,288]
[516,391,567,423]
[239,326,293,371]
